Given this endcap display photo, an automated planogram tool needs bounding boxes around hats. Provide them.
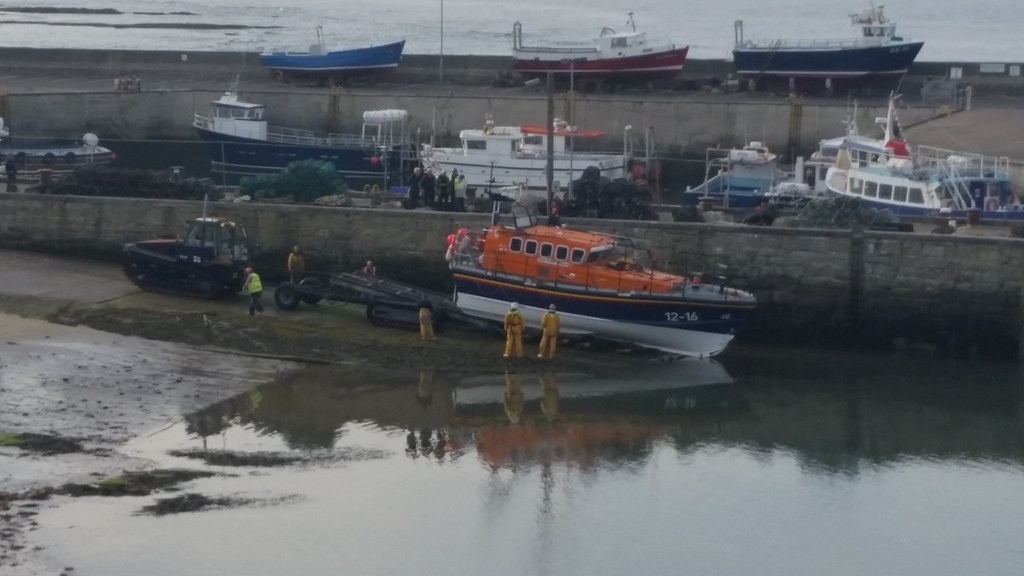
[550,303,556,310]
[451,168,457,175]
[367,261,373,266]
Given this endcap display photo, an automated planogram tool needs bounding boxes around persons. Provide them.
[369,155,384,172]
[406,165,467,213]
[242,267,266,318]
[537,303,561,361]
[417,291,438,342]
[502,301,526,358]
[288,245,305,284]
[4,154,18,193]
[361,259,376,276]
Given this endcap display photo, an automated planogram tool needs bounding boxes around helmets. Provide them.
[510,301,519,309]
[246,266,253,274]
[294,246,299,254]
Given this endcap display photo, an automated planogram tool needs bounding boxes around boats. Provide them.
[1,132,119,175]
[682,83,1024,232]
[443,190,760,359]
[730,0,928,95]
[417,106,670,209]
[509,10,692,81]
[452,343,739,469]
[119,190,253,300]
[258,24,408,82]
[191,73,425,193]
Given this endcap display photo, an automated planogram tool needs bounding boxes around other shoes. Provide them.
[422,336,426,340]
[538,353,543,357]
[549,354,554,359]
[430,336,436,340]
[516,353,524,357]
[503,353,510,357]
